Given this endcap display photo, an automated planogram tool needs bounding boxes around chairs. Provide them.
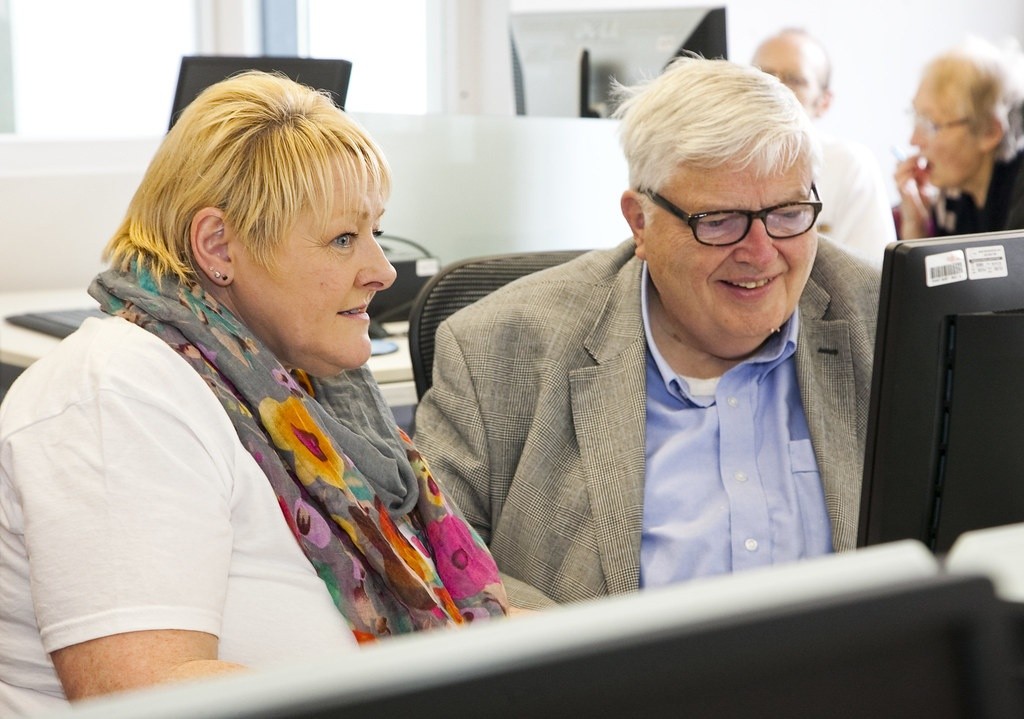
[409,251,588,402]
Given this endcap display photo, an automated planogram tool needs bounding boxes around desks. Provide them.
[0,291,414,383]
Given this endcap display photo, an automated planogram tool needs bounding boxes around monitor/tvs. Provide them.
[49,541,993,719]
[503,6,727,120]
[855,230,1024,554]
[167,56,353,138]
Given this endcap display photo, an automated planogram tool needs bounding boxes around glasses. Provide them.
[638,180,823,247]
[917,117,974,138]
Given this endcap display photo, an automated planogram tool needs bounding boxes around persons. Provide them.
[892,51,1024,242]
[1,67,539,719]
[403,30,885,608]
